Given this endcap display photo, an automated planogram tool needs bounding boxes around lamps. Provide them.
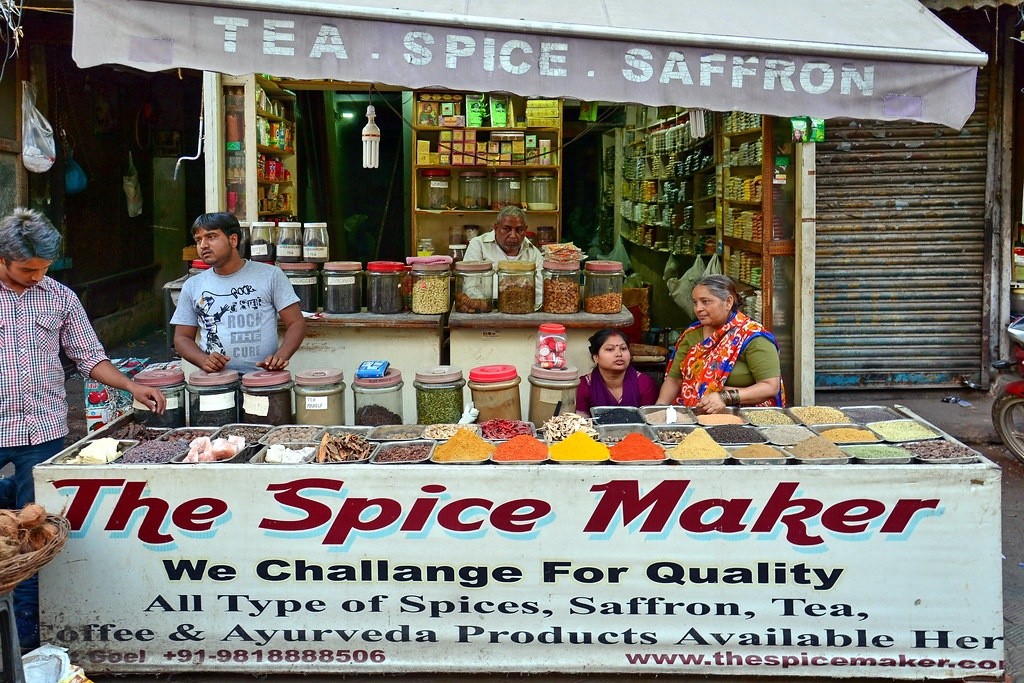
[335,110,354,121]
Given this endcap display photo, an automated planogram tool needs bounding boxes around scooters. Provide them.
[991,315,1024,465]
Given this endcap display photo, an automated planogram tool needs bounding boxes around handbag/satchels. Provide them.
[65,159,87,193]
[21,79,57,172]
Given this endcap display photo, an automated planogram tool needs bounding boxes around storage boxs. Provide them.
[441,103,455,116]
[525,108,559,118]
[455,102,461,115]
[226,111,293,213]
[417,130,558,165]
[527,99,558,108]
[411,92,562,257]
[524,118,559,127]
[438,115,465,127]
[417,101,440,126]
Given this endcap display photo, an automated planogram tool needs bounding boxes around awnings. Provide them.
[72,0,991,132]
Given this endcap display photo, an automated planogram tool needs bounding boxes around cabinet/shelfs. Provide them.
[222,74,298,222]
[600,110,793,402]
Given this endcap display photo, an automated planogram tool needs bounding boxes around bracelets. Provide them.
[717,389,740,407]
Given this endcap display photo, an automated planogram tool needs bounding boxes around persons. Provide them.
[655,274,786,414]
[793,129,803,142]
[575,328,660,419]
[0,206,167,683]
[174,212,307,374]
[463,206,546,304]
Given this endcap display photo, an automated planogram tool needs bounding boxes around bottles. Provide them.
[420,168,557,212]
[133,322,580,428]
[238,221,624,315]
